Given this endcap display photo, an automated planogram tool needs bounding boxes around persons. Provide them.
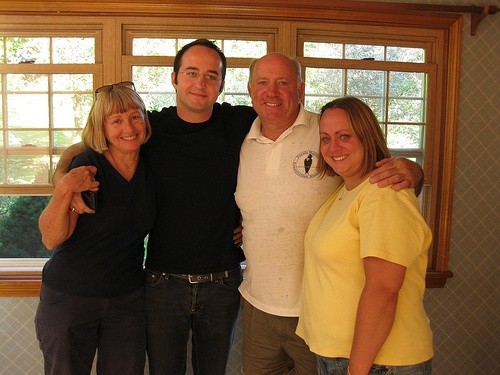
[35,81,167,375]
[234,51,424,375]
[293,97,435,375]
[52,38,259,375]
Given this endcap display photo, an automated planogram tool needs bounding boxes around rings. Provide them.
[92,178,96,182]
[71,208,75,211]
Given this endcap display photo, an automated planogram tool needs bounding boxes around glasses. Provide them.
[94,81,136,99]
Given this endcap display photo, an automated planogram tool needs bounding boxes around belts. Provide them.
[167,267,243,284]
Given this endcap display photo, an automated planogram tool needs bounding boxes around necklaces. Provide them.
[338,185,345,201]
